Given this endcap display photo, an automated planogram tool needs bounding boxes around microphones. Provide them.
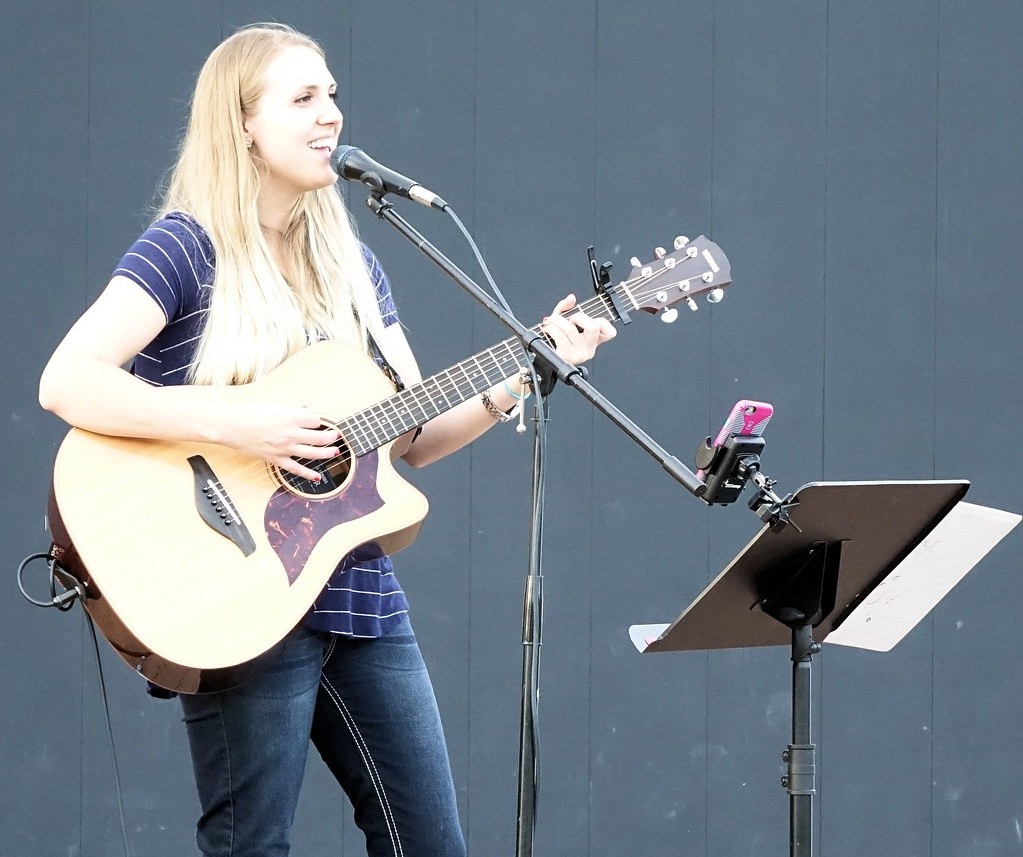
[329,145,449,213]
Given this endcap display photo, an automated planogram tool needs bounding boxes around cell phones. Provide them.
[694,400,773,484]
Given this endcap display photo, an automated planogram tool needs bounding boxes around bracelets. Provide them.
[503,381,531,401]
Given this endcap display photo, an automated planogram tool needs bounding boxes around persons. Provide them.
[37,28,618,857]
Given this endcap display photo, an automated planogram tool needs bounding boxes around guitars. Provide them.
[45,234,732,697]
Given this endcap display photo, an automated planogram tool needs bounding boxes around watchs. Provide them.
[480,388,520,423]
